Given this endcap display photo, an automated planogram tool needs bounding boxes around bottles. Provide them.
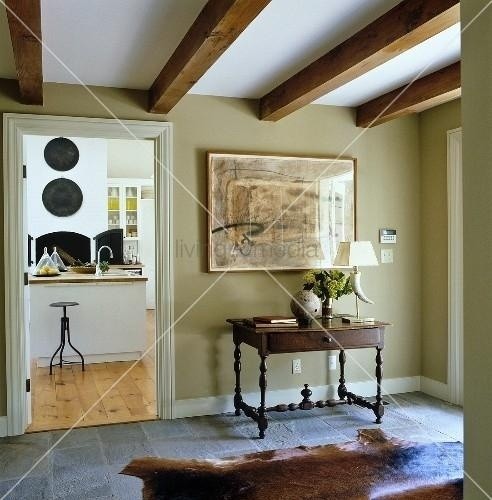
[123,245,137,266]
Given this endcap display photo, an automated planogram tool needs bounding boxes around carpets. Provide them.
[115,426,465,500]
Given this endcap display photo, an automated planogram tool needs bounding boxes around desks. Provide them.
[225,315,394,438]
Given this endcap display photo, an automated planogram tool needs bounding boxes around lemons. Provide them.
[39,266,57,275]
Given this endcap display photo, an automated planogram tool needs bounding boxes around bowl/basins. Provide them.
[66,266,105,275]
[141,186,155,200]
[126,231,137,238]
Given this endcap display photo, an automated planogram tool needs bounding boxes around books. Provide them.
[242,317,299,328]
[253,316,296,324]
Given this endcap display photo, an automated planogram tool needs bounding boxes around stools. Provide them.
[49,301,84,375]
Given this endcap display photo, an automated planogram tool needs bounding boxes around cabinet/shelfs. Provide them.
[108,185,138,262]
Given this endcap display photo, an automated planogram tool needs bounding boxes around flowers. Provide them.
[294,267,356,300]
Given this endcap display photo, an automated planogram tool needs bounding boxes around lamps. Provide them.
[333,240,379,325]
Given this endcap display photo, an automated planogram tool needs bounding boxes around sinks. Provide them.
[100,271,133,277]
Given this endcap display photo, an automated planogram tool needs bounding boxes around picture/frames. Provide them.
[205,149,358,274]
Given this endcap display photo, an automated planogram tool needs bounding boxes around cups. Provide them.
[126,215,137,225]
[108,215,119,225]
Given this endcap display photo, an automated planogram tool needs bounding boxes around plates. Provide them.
[59,269,67,272]
[32,273,62,277]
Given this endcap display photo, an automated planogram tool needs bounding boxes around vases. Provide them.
[321,297,334,319]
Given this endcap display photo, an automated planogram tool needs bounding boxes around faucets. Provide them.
[95,245,114,276]
